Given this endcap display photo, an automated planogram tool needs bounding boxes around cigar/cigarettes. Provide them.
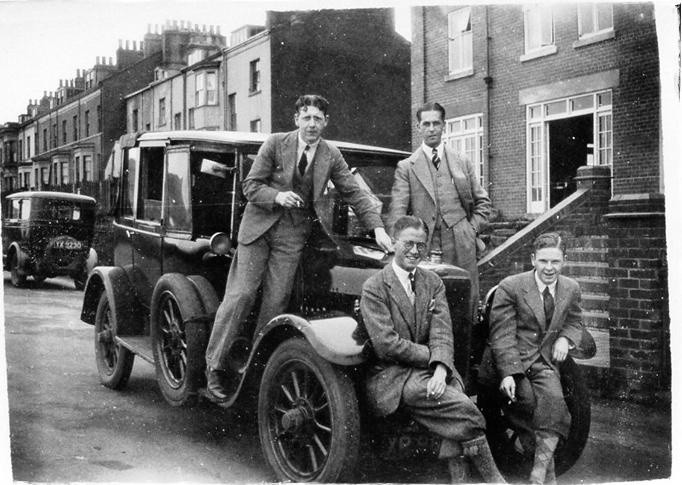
[431,391,435,396]
[297,200,300,207]
[508,399,512,405]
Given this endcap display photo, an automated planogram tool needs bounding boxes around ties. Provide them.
[431,147,441,169]
[542,286,555,330]
[298,146,310,176]
[409,272,416,291]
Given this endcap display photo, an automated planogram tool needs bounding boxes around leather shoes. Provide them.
[206,364,230,402]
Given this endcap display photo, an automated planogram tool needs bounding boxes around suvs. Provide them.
[1,190,99,291]
[80,129,591,483]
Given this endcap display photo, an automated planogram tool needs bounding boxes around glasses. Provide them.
[396,238,428,251]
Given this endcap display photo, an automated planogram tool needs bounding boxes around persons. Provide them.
[359,215,510,483]
[487,231,584,484]
[203,94,395,401]
[387,101,499,328]
